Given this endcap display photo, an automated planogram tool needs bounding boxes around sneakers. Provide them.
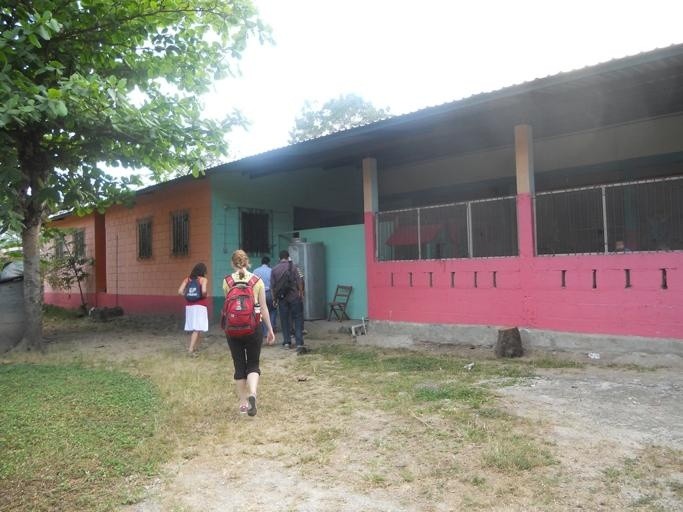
[273,326,310,350]
[185,349,200,359]
[239,403,248,413]
[248,392,257,417]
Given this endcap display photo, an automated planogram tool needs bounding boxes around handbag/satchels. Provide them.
[276,261,294,299]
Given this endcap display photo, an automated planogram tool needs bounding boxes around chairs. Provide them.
[326,283,353,321]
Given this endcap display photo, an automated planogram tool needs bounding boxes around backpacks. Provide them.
[219,275,261,337]
[185,277,202,301]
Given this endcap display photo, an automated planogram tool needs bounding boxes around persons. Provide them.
[253,255,279,339]
[270,249,311,350]
[612,239,631,252]
[177,262,211,359]
[220,249,275,419]
[288,256,308,338]
